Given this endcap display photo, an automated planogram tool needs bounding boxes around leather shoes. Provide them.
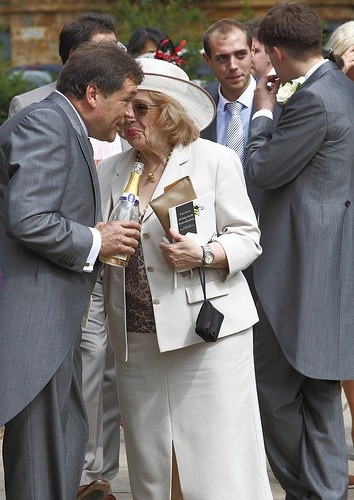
[77,478,117,500]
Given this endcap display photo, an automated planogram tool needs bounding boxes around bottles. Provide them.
[98,162,145,267]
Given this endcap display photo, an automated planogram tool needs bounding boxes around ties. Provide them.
[226,102,244,177]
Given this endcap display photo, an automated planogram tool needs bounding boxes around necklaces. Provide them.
[144,145,172,184]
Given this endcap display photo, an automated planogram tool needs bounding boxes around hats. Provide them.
[134,58,216,132]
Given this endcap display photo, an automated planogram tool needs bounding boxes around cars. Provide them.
[2,63,63,88]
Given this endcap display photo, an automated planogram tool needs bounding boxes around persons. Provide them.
[0,43,145,500]
[202,18,284,230]
[242,1,353,500]
[96,57,275,500]
[9,14,177,500]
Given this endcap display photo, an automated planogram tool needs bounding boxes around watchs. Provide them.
[202,244,214,268]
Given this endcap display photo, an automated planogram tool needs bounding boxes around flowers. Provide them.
[275,78,300,107]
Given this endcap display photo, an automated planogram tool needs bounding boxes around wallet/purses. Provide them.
[195,301,224,342]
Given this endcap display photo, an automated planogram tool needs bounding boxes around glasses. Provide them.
[132,103,158,116]
